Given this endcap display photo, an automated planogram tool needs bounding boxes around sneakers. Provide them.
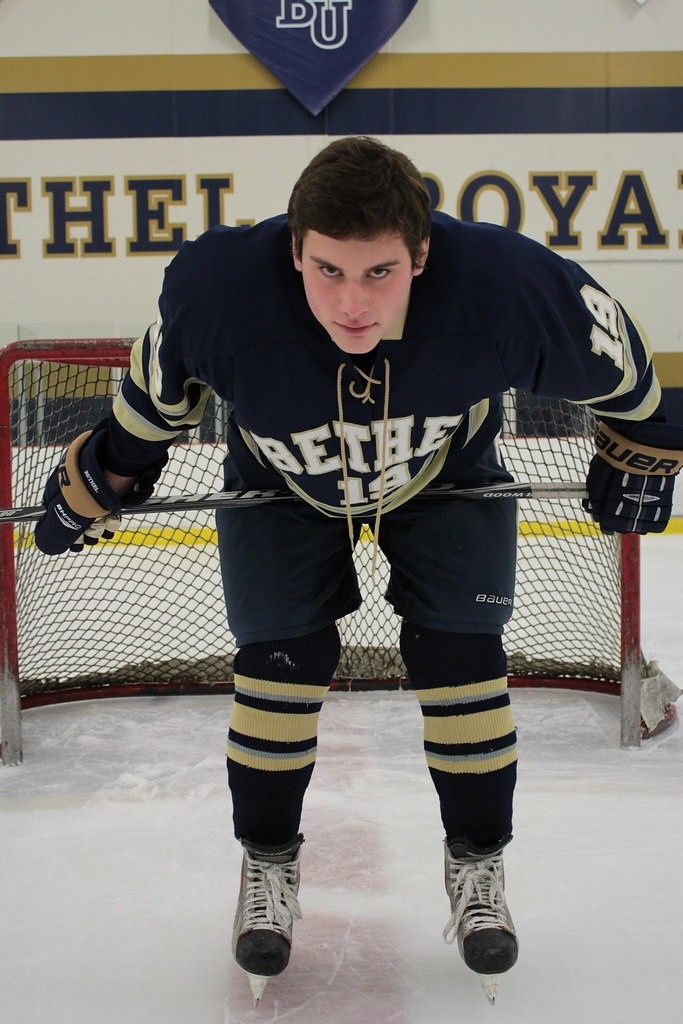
[232,833,306,1009]
[440,837,519,1005]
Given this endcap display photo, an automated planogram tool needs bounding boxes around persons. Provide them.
[32,136,683,1008]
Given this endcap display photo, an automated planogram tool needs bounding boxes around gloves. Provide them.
[582,416,683,537]
[33,427,168,557]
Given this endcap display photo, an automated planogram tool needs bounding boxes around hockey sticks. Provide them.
[0,481,589,524]
